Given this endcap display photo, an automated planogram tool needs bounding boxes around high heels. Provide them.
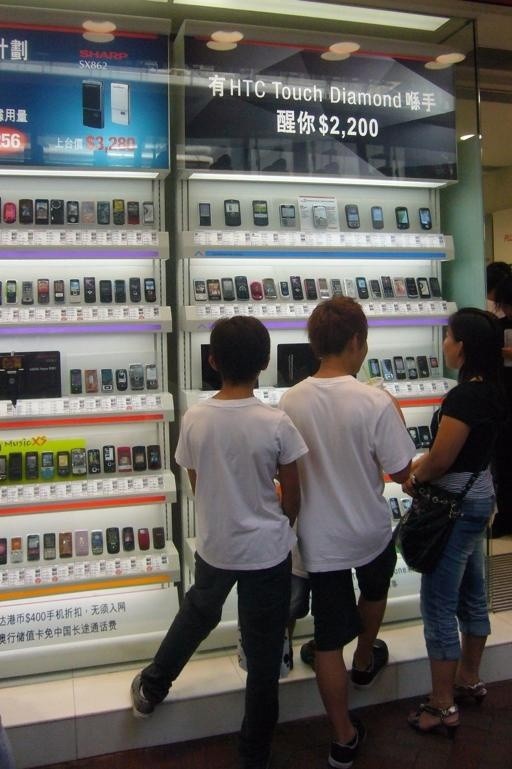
[406,703,462,737]
[453,679,489,712]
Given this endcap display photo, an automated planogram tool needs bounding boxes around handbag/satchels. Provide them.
[392,481,466,576]
[234,616,292,683]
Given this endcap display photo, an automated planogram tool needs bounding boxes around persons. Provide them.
[486,261,512,372]
[276,295,420,768]
[128,315,313,769]
[402,307,511,739]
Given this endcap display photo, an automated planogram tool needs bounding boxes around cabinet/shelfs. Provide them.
[197,20,463,659]
[2,0,186,680]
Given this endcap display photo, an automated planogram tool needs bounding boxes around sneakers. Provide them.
[129,666,160,720]
[289,639,318,672]
[484,511,512,539]
[349,636,391,686]
[326,716,369,769]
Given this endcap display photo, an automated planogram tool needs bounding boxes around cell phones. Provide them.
[368,355,440,381]
[193,276,441,301]
[0,197,154,224]
[406,426,433,449]
[0,526,165,565]
[0,276,156,305]
[388,497,412,519]
[82,80,103,128]
[70,363,159,393]
[199,200,432,230]
[0,444,161,481]
[110,83,131,126]
